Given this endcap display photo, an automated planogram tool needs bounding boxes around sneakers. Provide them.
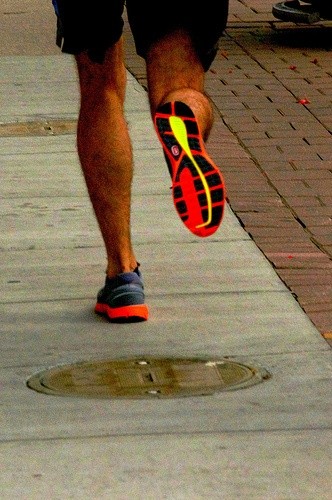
[155,102,226,238]
[95,263,151,322]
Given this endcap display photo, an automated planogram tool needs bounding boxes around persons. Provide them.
[49,0,231,323]
